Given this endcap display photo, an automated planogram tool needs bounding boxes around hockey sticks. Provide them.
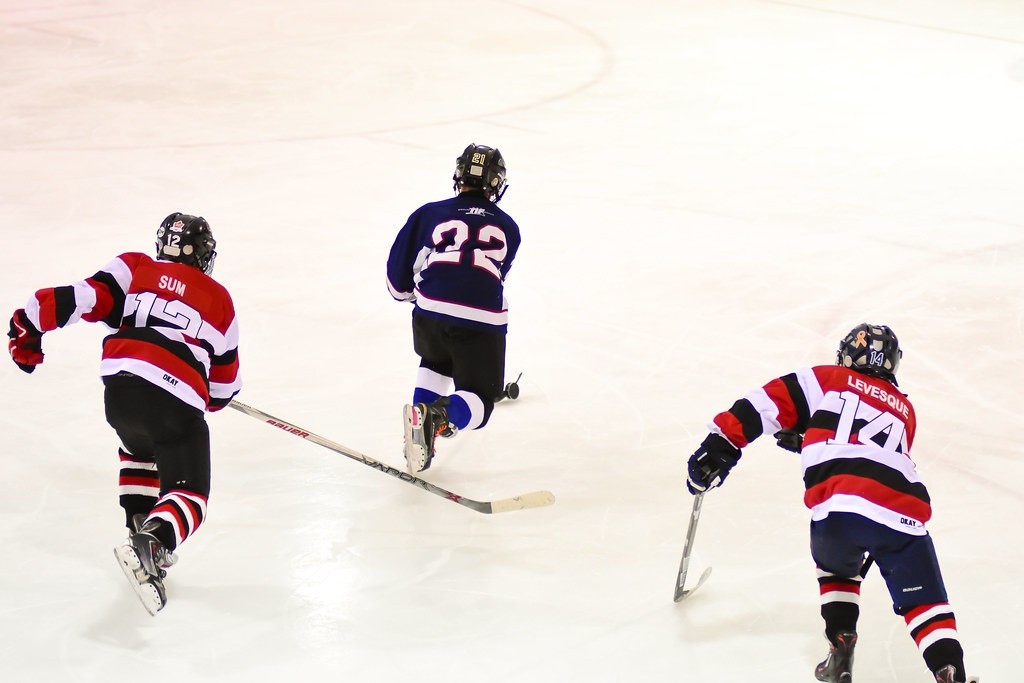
[672,494,713,601]
[229,400,555,515]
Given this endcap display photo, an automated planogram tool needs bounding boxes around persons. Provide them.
[687,323,978,683]
[386,143,521,472]
[8,212,242,612]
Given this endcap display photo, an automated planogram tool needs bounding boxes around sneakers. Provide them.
[114,545,164,616]
[128,515,179,567]
[431,399,459,440]
[403,402,449,473]
[935,664,976,683]
[816,632,858,683]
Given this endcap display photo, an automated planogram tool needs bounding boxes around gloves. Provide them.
[772,430,804,456]
[7,309,45,374]
[687,434,742,495]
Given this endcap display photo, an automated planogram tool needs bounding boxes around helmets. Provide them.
[453,142,508,204]
[837,323,903,389]
[156,212,217,276]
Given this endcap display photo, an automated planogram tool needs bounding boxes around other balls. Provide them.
[506,382,520,399]
